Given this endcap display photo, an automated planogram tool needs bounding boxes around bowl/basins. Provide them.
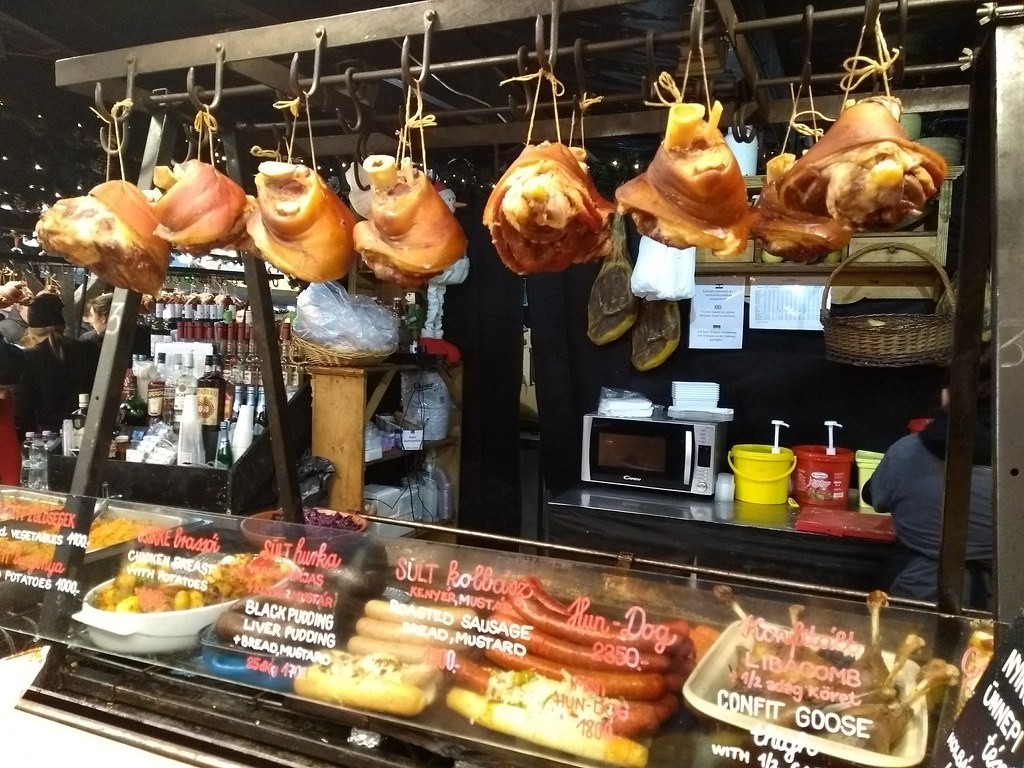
[671,381,719,408]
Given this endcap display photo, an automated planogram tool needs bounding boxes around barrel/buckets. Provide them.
[855,450,885,508]
[728,444,797,505]
[792,445,854,507]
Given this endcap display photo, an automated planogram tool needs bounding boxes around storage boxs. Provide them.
[365,484,421,538]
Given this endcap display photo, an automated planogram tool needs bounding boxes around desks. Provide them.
[546,488,897,593]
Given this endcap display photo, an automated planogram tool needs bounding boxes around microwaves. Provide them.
[581,412,726,496]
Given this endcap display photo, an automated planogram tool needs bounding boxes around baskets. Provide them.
[288,324,399,368]
[820,242,956,368]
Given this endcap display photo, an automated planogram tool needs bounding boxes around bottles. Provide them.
[110,280,265,472]
[20,429,62,492]
[71,394,89,456]
[272,302,304,386]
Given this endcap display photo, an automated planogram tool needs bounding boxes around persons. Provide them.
[75,293,114,387]
[0,288,114,441]
[861,345,996,611]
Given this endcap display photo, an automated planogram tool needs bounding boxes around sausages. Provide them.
[205,573,697,768]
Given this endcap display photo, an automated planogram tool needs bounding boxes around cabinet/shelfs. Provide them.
[303,363,464,547]
[693,164,965,269]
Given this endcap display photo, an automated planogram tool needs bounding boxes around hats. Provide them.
[28,293,66,325]
[929,360,990,422]
